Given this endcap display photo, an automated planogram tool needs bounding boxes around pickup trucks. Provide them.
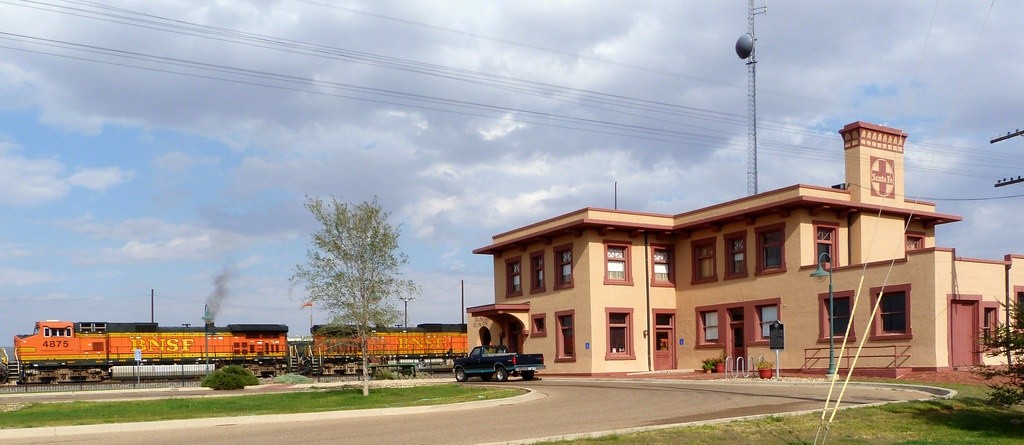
[452,345,546,382]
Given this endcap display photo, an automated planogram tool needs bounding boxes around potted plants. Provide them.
[702,355,727,373]
[756,357,775,378]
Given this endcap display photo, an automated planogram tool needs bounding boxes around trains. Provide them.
[0,288,468,386]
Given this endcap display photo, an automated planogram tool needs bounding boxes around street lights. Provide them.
[809,252,840,382]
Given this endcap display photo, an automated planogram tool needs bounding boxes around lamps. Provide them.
[644,330,648,336]
